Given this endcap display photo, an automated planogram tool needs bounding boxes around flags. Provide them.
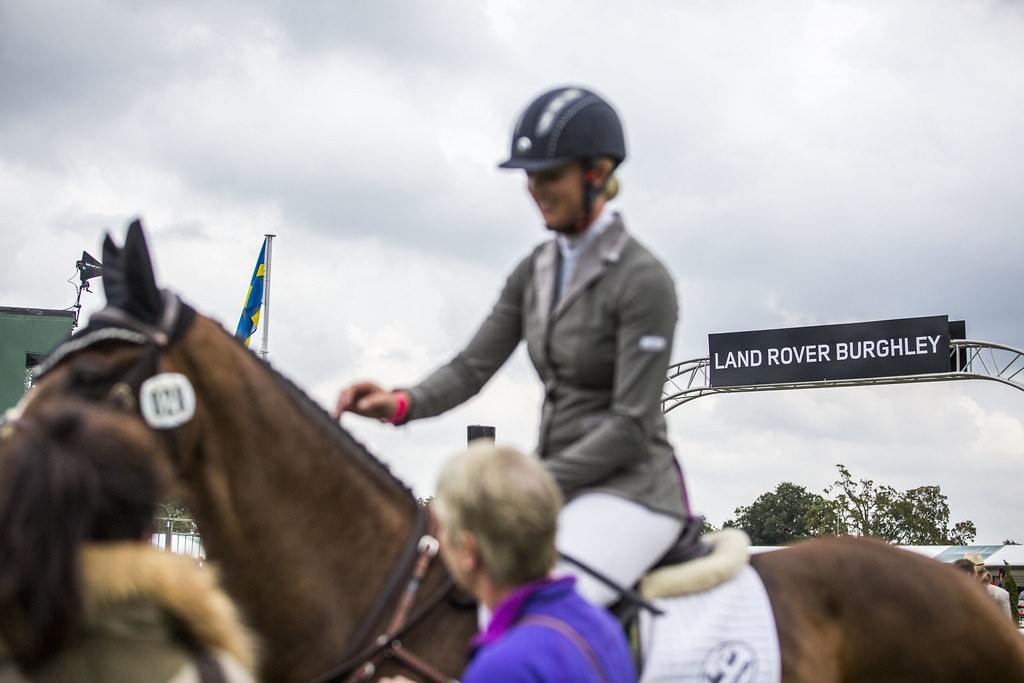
[235,233,276,366]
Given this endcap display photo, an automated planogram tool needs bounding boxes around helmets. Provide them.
[497,87,626,170]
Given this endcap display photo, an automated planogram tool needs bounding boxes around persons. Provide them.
[435,441,638,678]
[333,83,694,612]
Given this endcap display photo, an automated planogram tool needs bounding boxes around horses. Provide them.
[1,216,1024,683]
